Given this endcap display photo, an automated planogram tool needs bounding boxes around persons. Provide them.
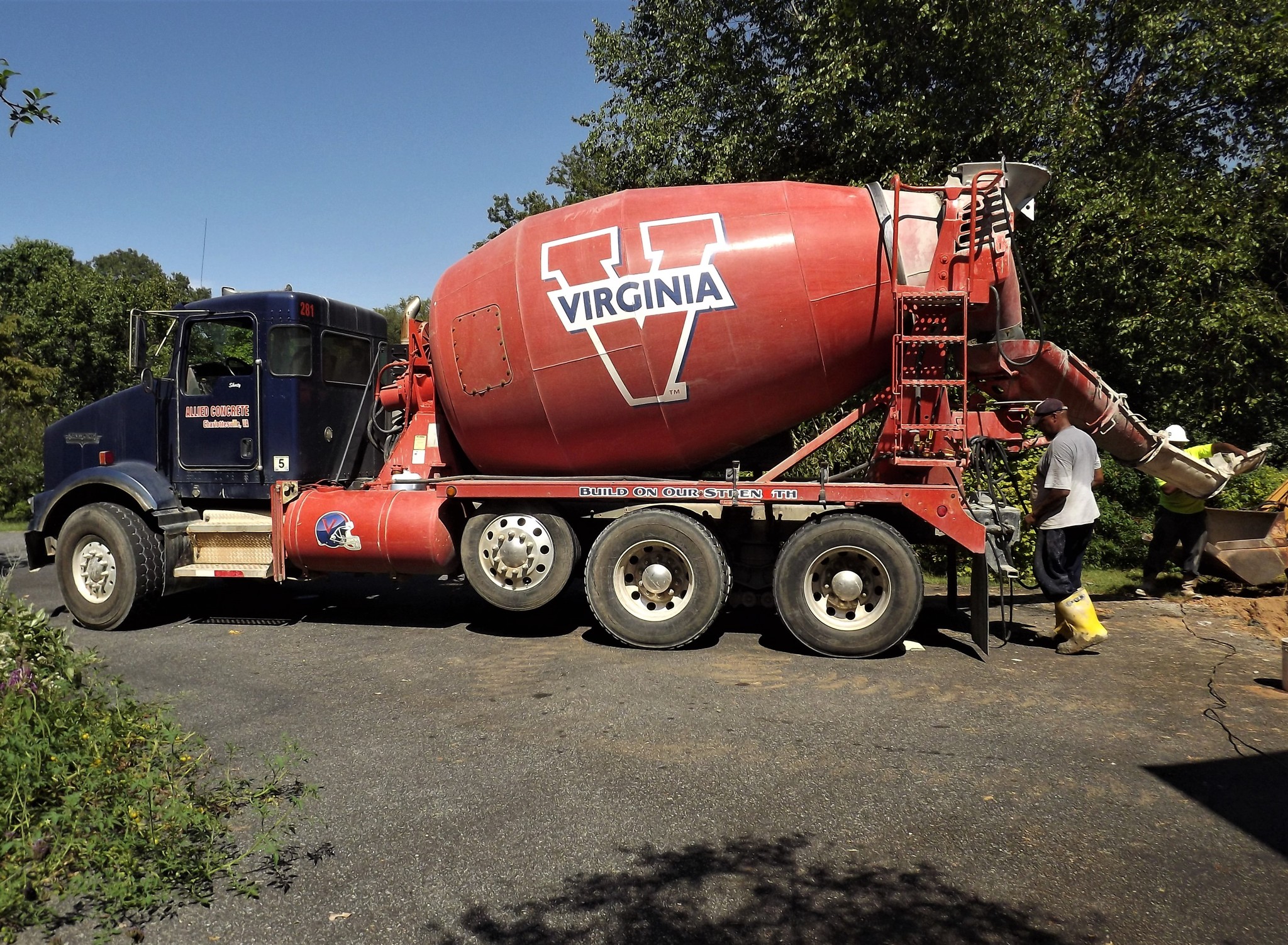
[1022,398,1109,654]
[1135,425,1248,599]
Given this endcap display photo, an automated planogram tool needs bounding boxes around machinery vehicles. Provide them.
[21,155,1278,662]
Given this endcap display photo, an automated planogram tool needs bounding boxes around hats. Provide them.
[1027,397,1069,425]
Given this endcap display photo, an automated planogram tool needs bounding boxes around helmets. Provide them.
[1165,424,1190,443]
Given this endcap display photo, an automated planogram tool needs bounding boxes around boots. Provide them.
[1035,598,1072,646]
[1135,571,1157,597]
[1177,574,1204,599]
[1056,587,1110,654]
